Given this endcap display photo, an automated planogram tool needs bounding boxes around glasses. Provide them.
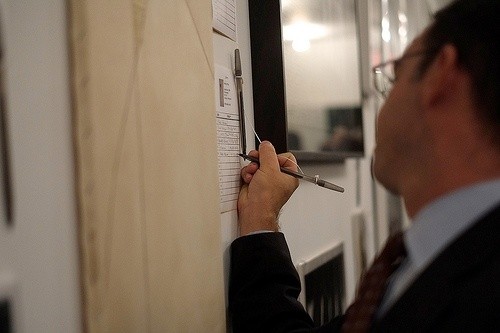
[371,42,448,99]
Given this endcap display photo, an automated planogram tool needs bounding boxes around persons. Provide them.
[228,0,500,333]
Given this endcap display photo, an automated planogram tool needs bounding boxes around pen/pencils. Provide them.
[237,152,345,195]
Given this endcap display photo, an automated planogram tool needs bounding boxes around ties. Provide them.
[340,228,410,332]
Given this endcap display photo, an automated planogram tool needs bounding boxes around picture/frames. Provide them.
[350,211,369,290]
[374,177,409,257]
[299,241,350,326]
